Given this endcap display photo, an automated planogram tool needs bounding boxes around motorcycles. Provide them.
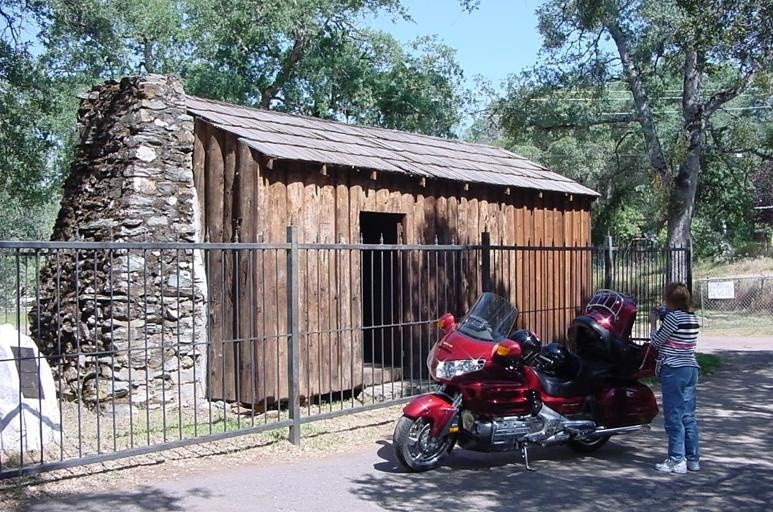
[392,291,660,473]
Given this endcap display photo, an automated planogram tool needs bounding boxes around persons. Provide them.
[648,281,702,475]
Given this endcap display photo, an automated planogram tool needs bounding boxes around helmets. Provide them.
[511,330,541,364]
[535,344,570,378]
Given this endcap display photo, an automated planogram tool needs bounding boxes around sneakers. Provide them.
[655,458,700,474]
